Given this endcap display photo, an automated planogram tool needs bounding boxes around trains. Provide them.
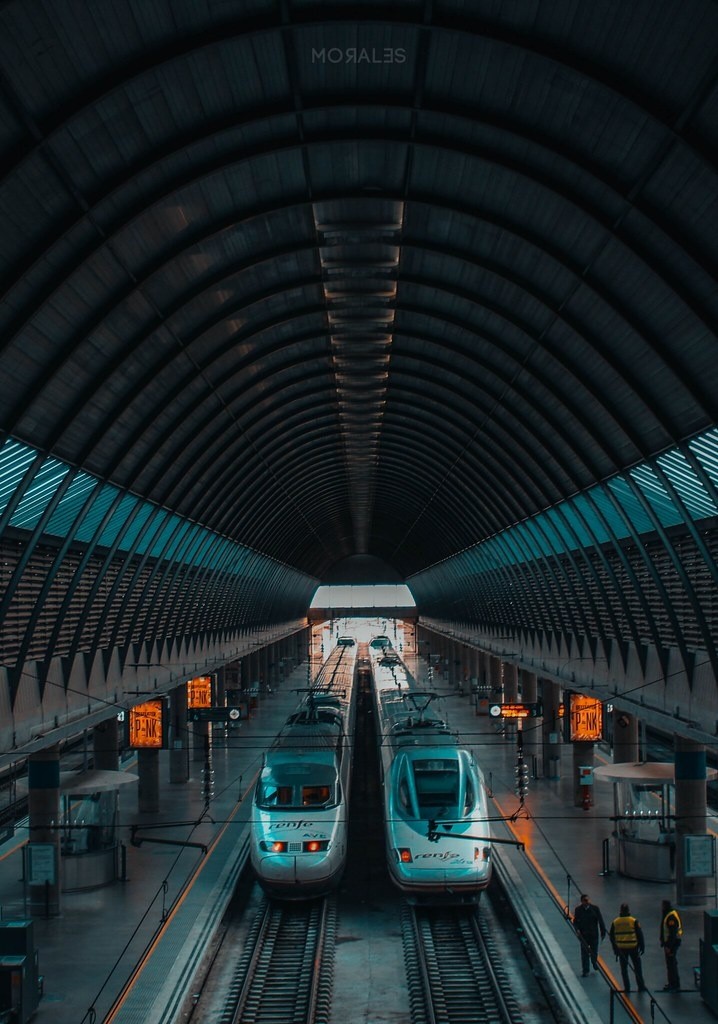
[368,635,492,909]
[249,633,359,902]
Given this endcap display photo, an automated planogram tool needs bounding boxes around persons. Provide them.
[610,903,645,992]
[660,900,683,991]
[573,895,606,977]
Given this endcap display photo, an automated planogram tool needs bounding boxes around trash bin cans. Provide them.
[549,757,560,777]
[475,694,489,715]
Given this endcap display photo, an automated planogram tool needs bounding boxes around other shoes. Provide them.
[625,988,630,993]
[582,972,590,978]
[664,983,680,992]
[593,964,599,970]
[638,986,646,992]
